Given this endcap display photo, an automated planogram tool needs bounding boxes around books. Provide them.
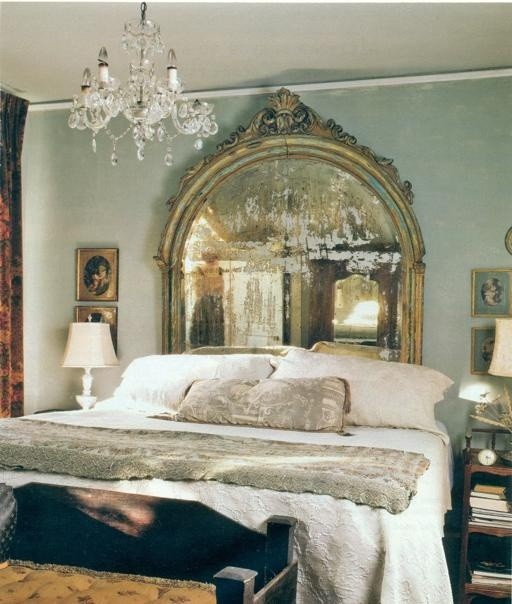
[468,483,512,529]
[464,560,512,599]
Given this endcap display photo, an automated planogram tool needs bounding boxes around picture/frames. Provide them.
[470,268,511,318]
[471,326,495,378]
[75,247,119,302]
[74,306,119,356]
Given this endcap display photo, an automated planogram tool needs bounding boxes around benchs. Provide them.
[0,484,298,604]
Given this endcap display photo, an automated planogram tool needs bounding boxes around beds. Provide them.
[2,347,455,604]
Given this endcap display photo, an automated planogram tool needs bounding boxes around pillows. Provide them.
[115,354,271,422]
[273,346,454,433]
[175,377,348,435]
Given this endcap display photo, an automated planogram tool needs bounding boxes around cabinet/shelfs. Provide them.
[460,427,512,603]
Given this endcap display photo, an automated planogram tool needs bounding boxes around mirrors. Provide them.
[152,87,426,367]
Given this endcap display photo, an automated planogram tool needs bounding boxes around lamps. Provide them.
[68,3,220,167]
[62,322,122,410]
[488,316,512,379]
